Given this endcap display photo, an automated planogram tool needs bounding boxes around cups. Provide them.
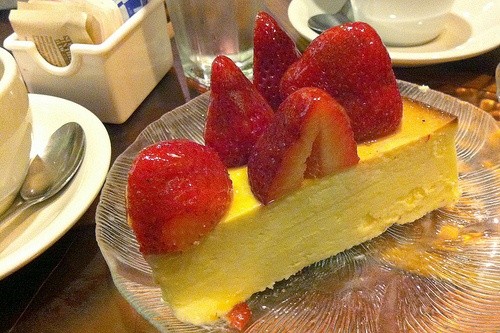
[0,48,34,218]
[165,0,290,90]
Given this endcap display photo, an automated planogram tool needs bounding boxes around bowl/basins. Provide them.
[350,0,454,46]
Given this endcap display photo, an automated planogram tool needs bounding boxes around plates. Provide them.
[288,0,500,69]
[0,93,113,279]
[95,78,500,333]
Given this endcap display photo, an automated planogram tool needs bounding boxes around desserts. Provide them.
[126,12,459,328]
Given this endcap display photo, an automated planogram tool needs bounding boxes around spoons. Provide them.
[307,0,351,34]
[0,122,88,233]
